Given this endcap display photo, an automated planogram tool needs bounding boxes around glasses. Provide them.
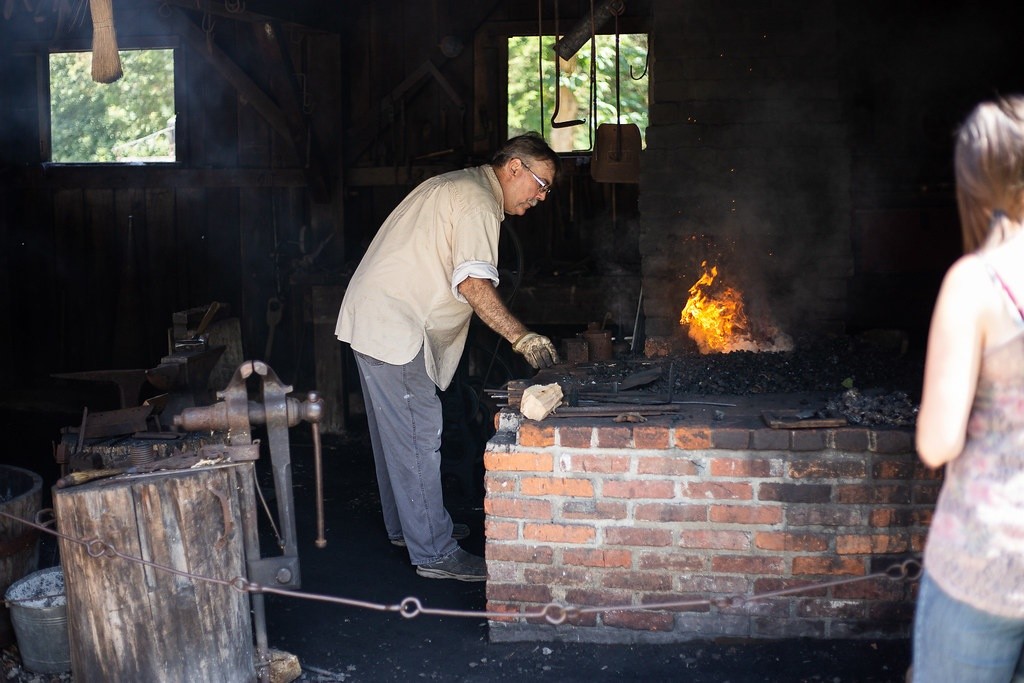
[521,160,552,196]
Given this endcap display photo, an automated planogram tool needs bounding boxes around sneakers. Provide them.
[417,544,487,584]
[390,522,471,545]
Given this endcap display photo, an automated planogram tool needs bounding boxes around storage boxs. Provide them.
[4,564,73,675]
[0,463,44,638]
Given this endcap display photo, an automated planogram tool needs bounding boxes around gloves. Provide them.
[513,331,560,370]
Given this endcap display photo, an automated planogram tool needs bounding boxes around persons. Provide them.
[337,131,560,585]
[902,95,1023,683]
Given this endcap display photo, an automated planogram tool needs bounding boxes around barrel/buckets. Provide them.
[0,465,44,592]
[4,565,73,674]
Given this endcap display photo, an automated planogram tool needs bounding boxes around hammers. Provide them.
[175,301,222,352]
